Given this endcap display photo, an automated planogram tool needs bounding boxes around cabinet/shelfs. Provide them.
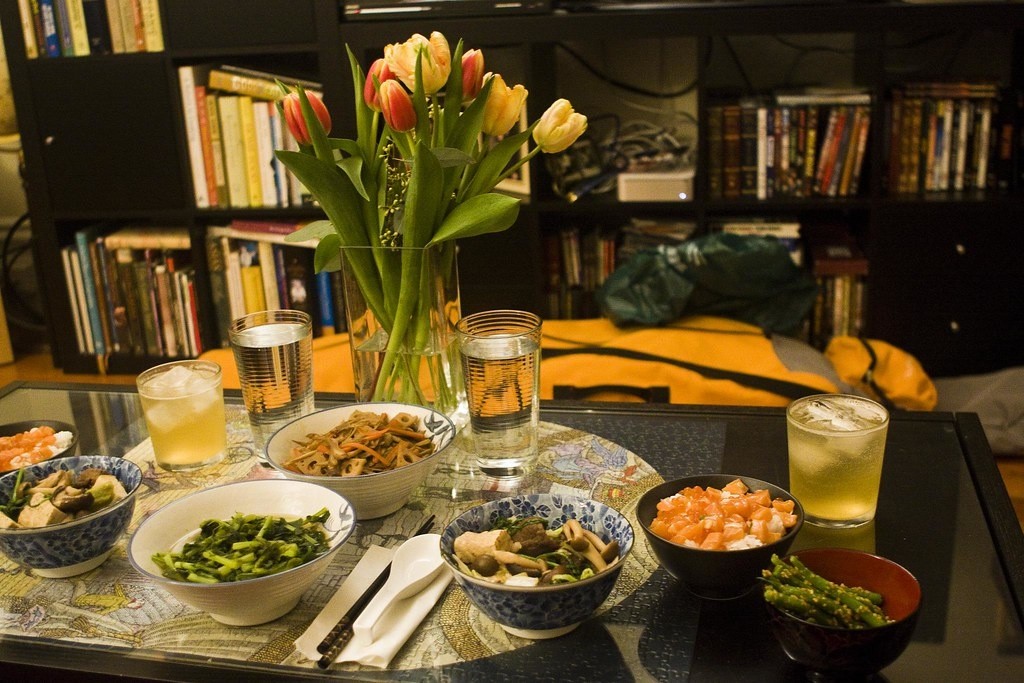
[0,0,1024,383]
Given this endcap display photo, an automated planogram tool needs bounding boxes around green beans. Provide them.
[761,554,890,629]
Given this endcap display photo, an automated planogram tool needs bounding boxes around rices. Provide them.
[11,427,73,469]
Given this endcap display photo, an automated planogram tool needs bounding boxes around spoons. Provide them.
[352,533,445,646]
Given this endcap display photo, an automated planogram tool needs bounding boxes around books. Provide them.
[176,60,326,209]
[58,220,351,357]
[883,82,1024,191]
[543,219,871,354]
[17,0,166,59]
[699,84,871,199]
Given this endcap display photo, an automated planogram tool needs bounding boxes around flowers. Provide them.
[271,31,587,409]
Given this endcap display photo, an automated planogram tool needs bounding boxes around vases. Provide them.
[339,243,472,436]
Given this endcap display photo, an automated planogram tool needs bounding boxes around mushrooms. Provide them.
[473,516,619,586]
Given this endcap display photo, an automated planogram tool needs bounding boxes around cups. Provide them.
[786,393,889,530]
[136,359,230,472]
[229,309,316,464]
[455,309,545,468]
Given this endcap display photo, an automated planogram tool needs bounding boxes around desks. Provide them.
[0,383,1024,683]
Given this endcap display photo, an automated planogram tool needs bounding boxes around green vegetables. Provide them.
[152,507,328,583]
[0,464,116,517]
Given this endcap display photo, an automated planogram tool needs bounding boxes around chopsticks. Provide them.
[316,514,436,672]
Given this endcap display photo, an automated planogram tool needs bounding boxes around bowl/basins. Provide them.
[127,479,358,626]
[440,493,636,640]
[0,420,79,476]
[263,402,456,521]
[765,547,923,676]
[0,455,142,580]
[637,474,806,601]
